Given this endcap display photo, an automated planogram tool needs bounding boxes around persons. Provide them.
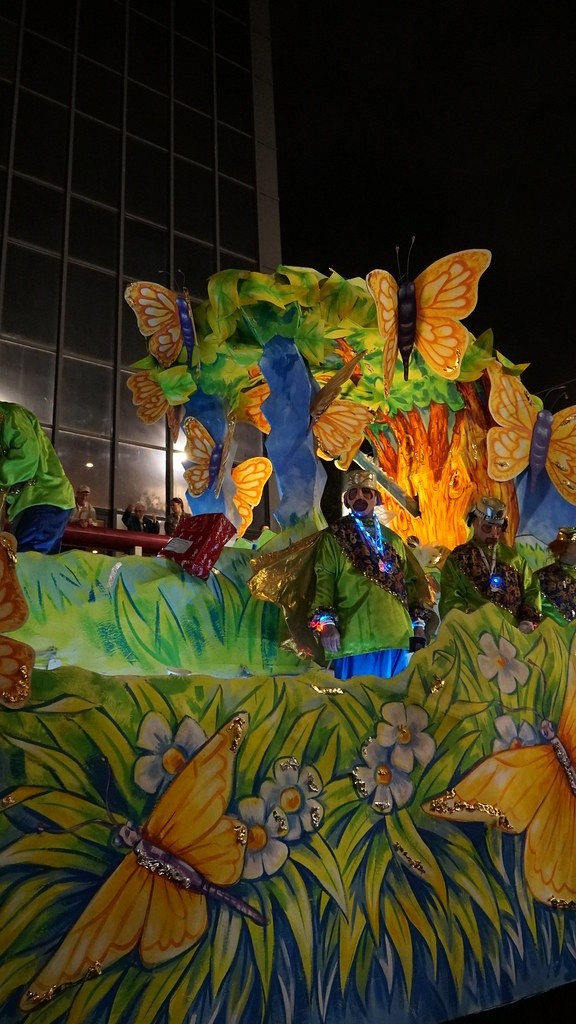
[0,400,76,554]
[439,494,543,634]
[309,470,427,680]
[122,503,161,535]
[69,484,96,527]
[531,527,576,624]
[165,498,192,536]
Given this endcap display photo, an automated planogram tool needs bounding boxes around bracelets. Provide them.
[320,616,337,626]
[412,618,426,631]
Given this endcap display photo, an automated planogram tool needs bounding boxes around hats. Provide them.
[475,496,508,524]
[557,526,576,541]
[77,484,90,493]
[344,470,377,492]
